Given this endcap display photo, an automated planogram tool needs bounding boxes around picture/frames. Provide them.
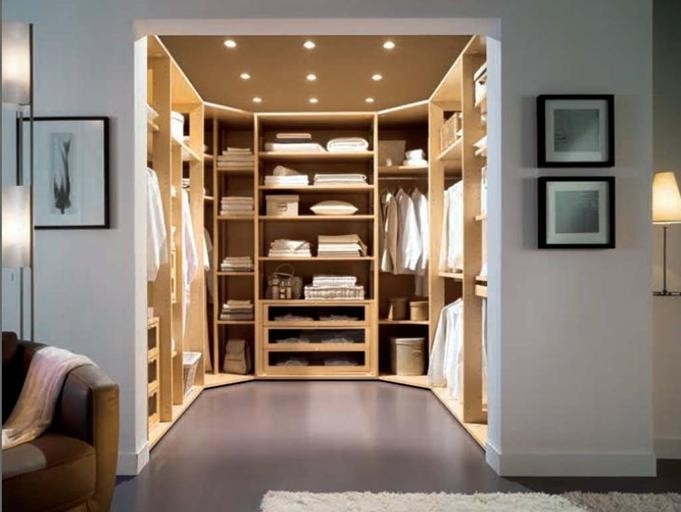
[16,115,108,230]
[536,175,616,250]
[536,93,616,169]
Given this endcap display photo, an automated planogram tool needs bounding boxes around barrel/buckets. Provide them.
[390,337,425,376]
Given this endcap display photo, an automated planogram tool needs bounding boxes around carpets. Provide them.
[257,489,679,512]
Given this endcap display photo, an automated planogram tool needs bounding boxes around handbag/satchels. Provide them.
[264,263,303,299]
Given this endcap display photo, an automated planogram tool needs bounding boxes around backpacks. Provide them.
[223,337,252,374]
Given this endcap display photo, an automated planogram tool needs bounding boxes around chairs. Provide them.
[1,331,119,512]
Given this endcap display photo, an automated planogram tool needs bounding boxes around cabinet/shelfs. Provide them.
[169,57,203,426]
[434,56,462,420]
[203,103,255,386]
[463,33,488,449]
[255,113,374,378]
[378,100,431,384]
[147,33,173,448]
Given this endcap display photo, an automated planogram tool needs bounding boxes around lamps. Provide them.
[651,172,681,298]
[2,19,33,341]
[1,186,36,343]
